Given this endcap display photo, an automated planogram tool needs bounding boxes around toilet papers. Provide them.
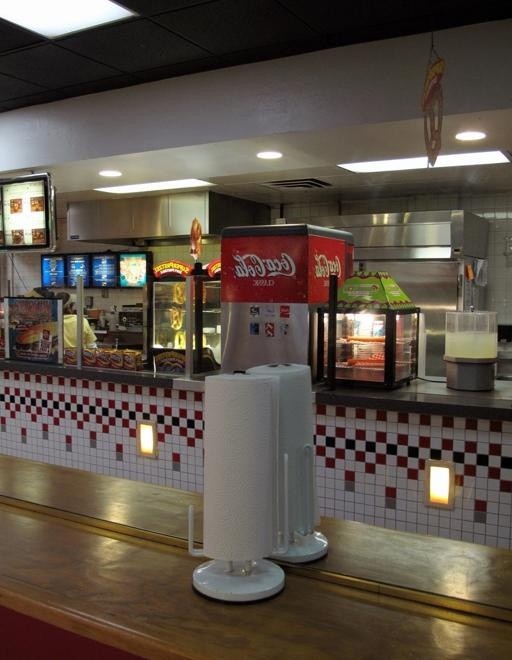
[245,360,322,545]
[202,372,279,561]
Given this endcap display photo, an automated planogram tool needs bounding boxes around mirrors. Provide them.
[1,0,512,627]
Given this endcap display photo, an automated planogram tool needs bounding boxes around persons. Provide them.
[43,330,51,340]
[56,291,97,350]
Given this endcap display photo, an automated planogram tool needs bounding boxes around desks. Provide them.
[0,499,512,660]
[0,450,512,628]
